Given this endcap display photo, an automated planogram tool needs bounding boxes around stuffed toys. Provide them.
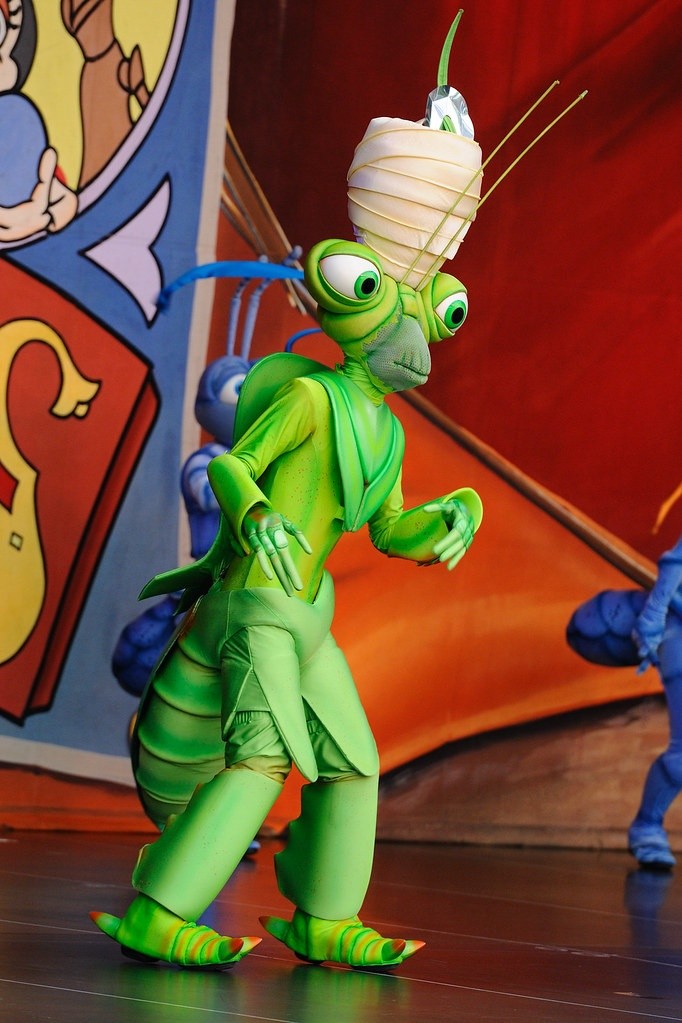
[90,118,482,968]
[565,529,682,868]
[110,245,285,700]
